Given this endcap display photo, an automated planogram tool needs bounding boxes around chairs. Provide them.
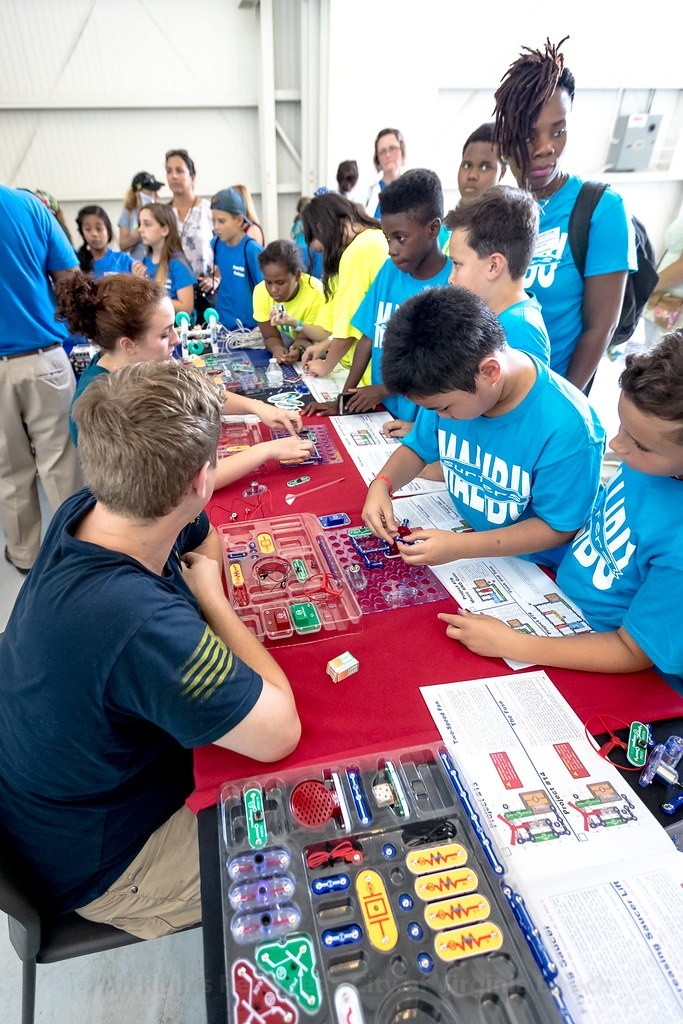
[0,824,204,1024]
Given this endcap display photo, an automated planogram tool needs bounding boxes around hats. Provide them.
[210,189,254,228]
[132,173,166,192]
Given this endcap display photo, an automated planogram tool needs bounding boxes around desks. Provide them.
[171,337,683,818]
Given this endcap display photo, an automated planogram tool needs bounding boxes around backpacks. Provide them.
[569,180,659,343]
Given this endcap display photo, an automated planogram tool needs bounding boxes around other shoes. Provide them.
[6,546,30,574]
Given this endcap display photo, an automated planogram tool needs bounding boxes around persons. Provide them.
[438,329,683,695]
[0,359,302,941]
[0,34,639,575]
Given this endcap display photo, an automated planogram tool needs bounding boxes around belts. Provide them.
[0,342,61,362]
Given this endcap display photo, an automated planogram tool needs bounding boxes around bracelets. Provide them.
[368,475,394,498]
[294,321,303,332]
